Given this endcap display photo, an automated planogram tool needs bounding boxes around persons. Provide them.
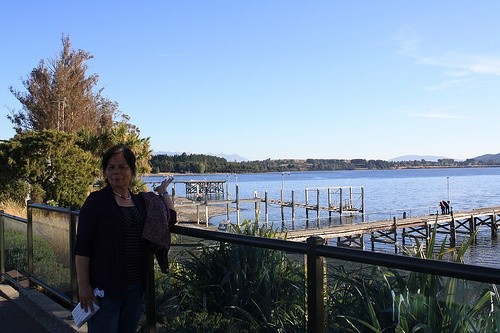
[441,203,445,215]
[74,144,177,333]
[446,202,449,214]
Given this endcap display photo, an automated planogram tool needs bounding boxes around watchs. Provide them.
[159,191,168,197]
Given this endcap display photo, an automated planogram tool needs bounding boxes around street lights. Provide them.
[281,171,292,203]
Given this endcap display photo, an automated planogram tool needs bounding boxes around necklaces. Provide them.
[113,190,131,200]
